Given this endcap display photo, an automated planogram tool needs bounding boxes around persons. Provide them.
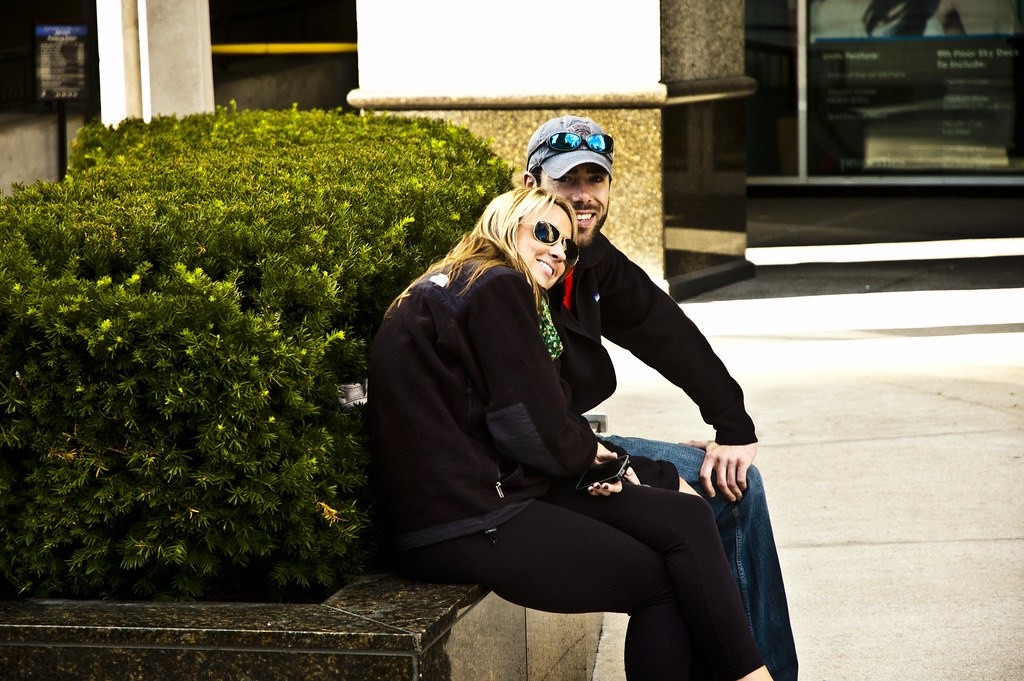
[864,0,963,38]
[372,190,773,681]
[523,117,800,681]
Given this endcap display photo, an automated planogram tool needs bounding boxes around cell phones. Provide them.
[576,455,632,491]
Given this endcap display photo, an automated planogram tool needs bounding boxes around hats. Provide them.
[527,116,613,181]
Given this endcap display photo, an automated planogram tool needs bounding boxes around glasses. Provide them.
[527,132,613,155]
[523,220,580,267]
